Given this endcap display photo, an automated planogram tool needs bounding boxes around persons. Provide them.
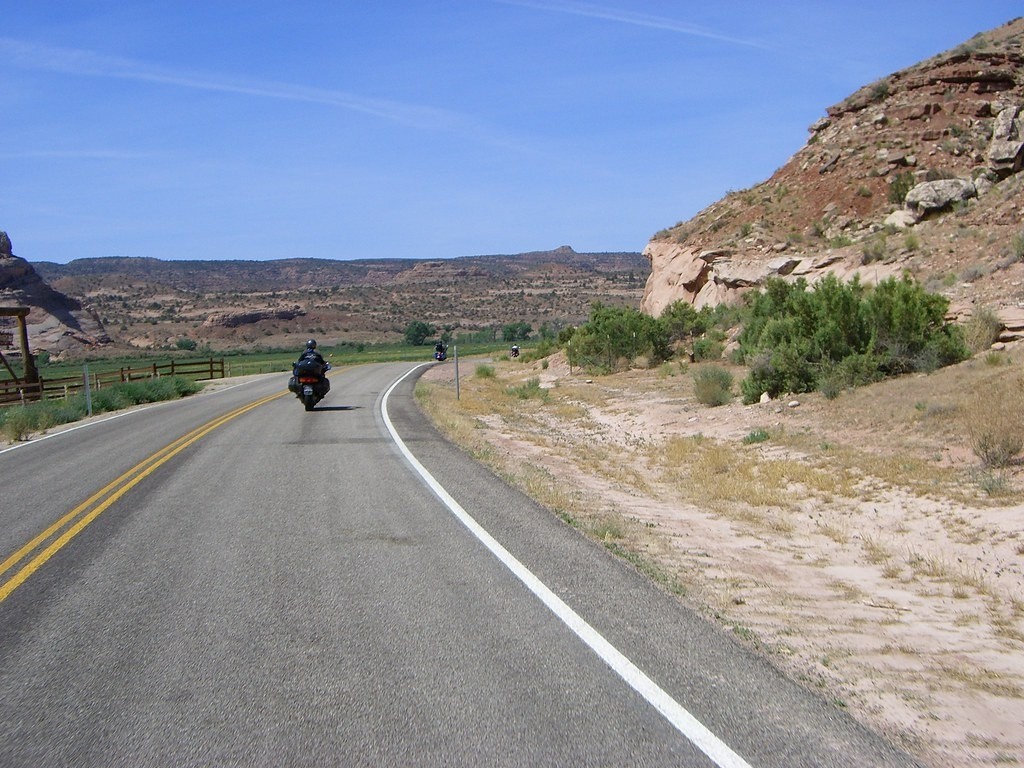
[294,339,331,400]
[436,341,445,352]
[511,343,519,356]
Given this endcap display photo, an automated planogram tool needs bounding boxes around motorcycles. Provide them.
[512,347,520,357]
[291,362,331,412]
[434,345,449,361]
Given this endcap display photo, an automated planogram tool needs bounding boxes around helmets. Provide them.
[306,339,316,348]
